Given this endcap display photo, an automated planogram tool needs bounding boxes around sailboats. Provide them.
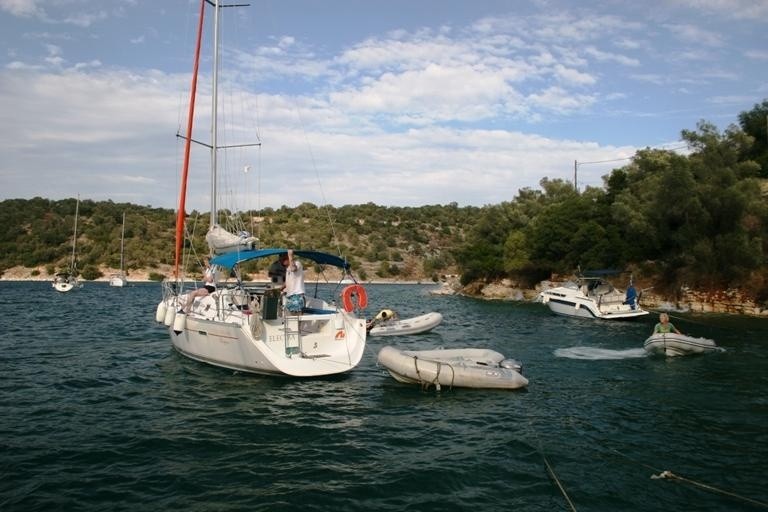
[52,191,85,294]
[109,211,128,288]
[155,0,368,380]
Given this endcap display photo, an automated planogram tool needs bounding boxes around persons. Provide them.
[267,254,286,282]
[366,309,397,331]
[278,248,306,332]
[176,256,217,314]
[652,313,681,335]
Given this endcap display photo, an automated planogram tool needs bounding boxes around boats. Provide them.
[643,332,727,359]
[376,345,530,390]
[369,311,444,338]
[536,277,650,321]
